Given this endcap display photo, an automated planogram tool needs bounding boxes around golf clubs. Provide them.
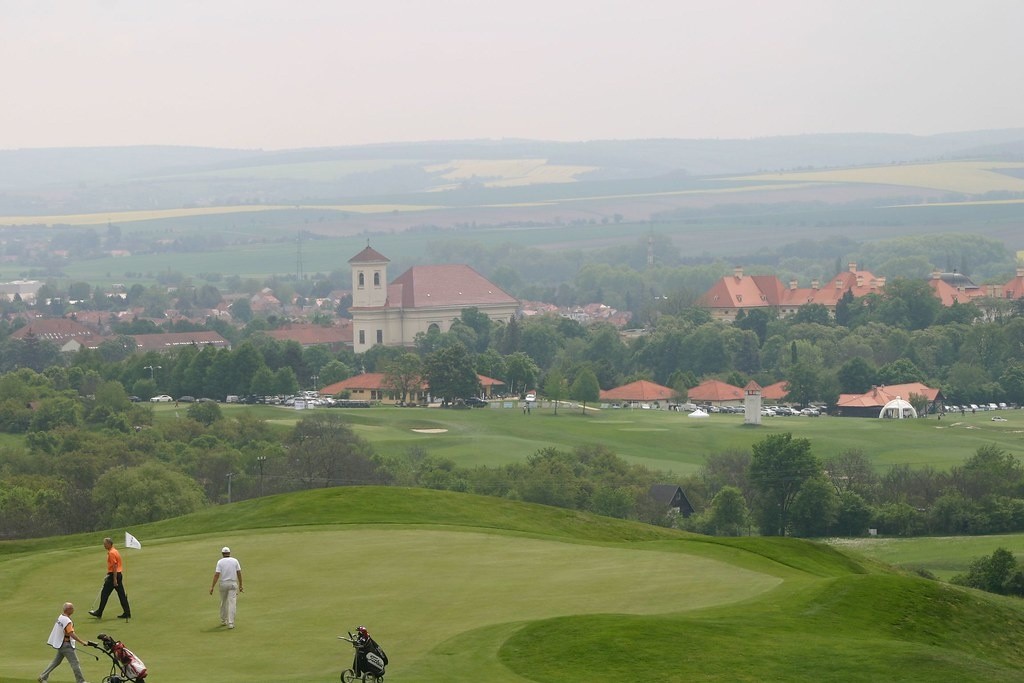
[337,625,368,644]
[73,645,100,661]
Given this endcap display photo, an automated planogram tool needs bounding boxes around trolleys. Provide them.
[84,640,147,683]
[338,628,387,683]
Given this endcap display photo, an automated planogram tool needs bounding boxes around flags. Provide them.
[125,533,141,549]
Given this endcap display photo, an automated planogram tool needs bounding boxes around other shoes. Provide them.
[38,677,47,683]
[89,611,101,618]
[222,620,227,625]
[117,612,131,618]
[228,624,235,628]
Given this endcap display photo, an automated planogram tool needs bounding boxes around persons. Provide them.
[37,603,89,683]
[209,547,243,629]
[89,538,131,618]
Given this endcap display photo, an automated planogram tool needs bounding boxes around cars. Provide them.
[525,394,536,401]
[610,400,1024,422]
[127,390,335,411]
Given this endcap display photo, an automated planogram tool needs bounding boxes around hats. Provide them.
[222,547,230,553]
